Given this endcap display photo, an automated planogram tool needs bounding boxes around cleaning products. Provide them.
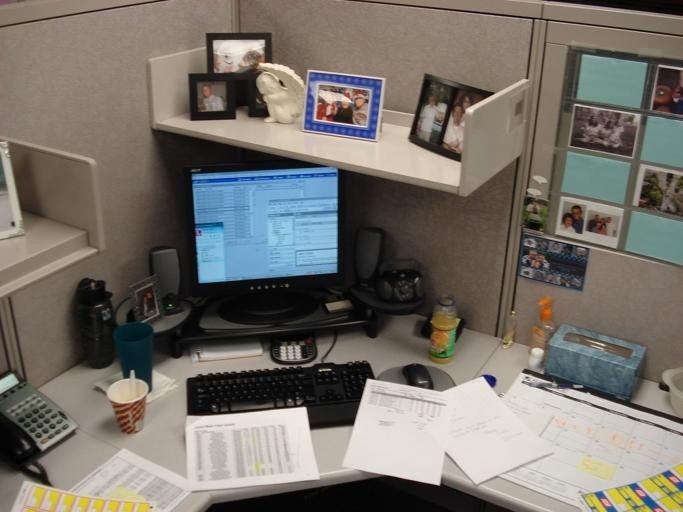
[529,295,558,355]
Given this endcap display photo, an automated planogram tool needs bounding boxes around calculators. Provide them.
[270,333,317,364]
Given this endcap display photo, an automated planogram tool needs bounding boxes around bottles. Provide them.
[500,311,517,349]
[74,278,116,368]
[428,295,460,365]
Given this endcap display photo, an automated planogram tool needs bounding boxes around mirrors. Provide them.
[149,244,182,300]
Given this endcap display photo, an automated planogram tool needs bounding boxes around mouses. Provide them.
[405,363,433,390]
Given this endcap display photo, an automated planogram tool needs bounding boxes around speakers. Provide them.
[148,246,182,314]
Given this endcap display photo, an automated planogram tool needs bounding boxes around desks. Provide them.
[0,296,501,512]
[381,340,683,512]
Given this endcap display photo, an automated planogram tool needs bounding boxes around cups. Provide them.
[112,321,155,394]
[105,378,150,435]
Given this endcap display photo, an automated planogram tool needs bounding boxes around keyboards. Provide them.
[187,360,379,430]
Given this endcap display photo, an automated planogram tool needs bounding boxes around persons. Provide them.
[459,95,471,127]
[333,95,353,123]
[416,94,445,143]
[574,115,626,149]
[526,250,545,269]
[571,204,584,234]
[350,92,369,127]
[588,213,618,239]
[443,105,463,154]
[559,212,577,236]
[202,83,224,112]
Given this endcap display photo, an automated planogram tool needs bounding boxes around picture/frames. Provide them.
[522,196,550,232]
[204,32,273,102]
[518,231,590,292]
[244,66,267,117]
[129,274,164,325]
[547,45,683,270]
[0,141,26,240]
[189,70,236,120]
[408,72,494,161]
[303,69,386,142]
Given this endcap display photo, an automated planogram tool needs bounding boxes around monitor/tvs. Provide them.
[186,165,350,326]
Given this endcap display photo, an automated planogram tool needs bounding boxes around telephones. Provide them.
[0,369,78,487]
